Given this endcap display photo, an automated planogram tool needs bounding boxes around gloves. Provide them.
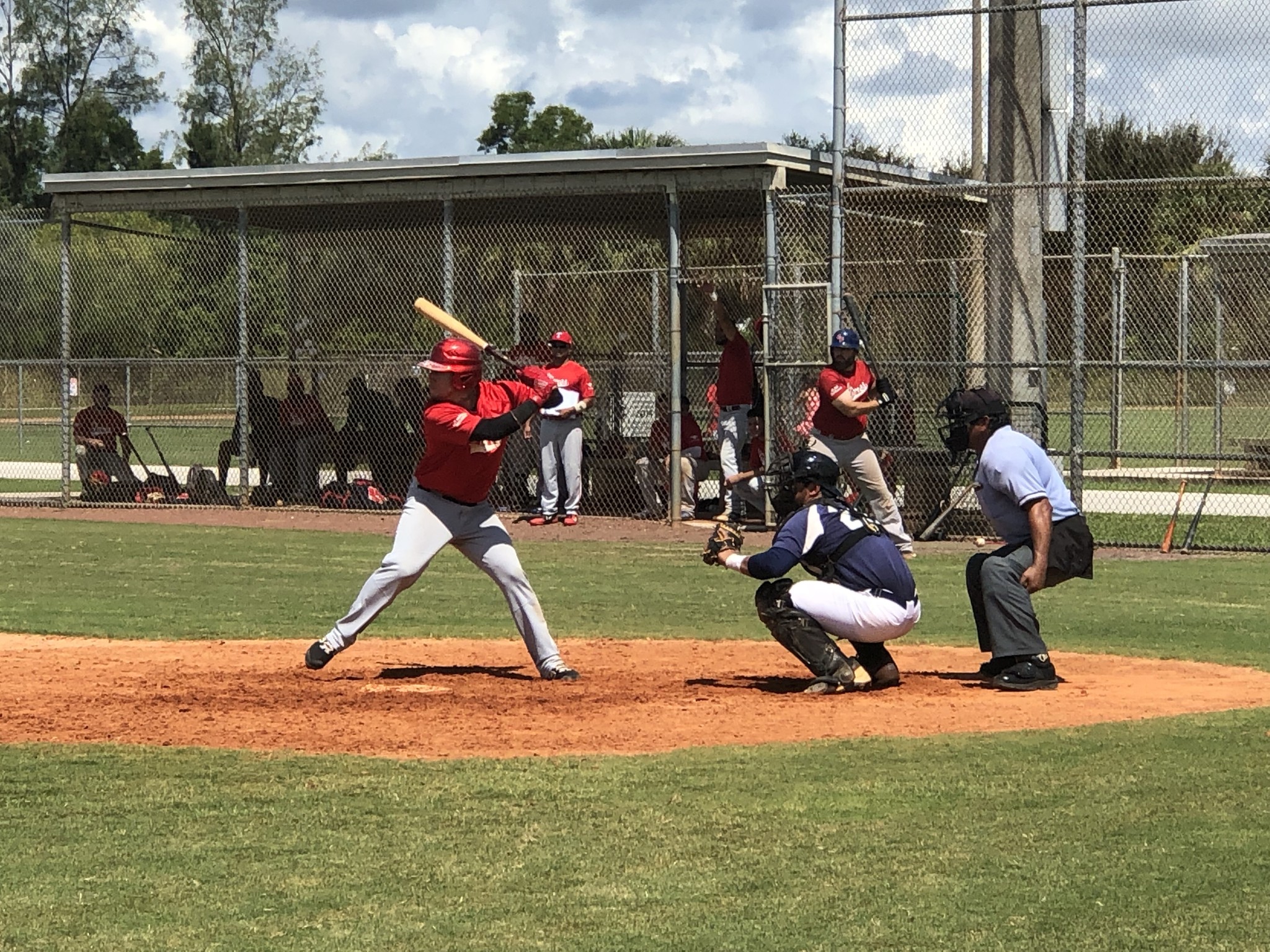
[875,377,895,406]
[528,372,558,405]
[515,364,559,392]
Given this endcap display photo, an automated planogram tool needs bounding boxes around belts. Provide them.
[416,483,477,508]
[540,413,583,420]
[820,429,864,441]
[869,587,921,609]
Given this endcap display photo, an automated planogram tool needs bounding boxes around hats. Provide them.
[786,451,843,498]
[943,388,1012,415]
[549,331,573,345]
[342,377,376,397]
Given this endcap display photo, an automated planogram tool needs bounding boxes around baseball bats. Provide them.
[1180,477,1215,555]
[122,432,150,477]
[414,297,523,372]
[1159,480,1188,554]
[146,426,177,483]
[843,293,881,379]
[913,451,977,541]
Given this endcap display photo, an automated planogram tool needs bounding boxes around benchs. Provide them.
[588,439,953,516]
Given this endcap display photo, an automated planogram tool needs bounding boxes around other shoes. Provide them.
[680,511,695,520]
[634,506,668,521]
[899,550,917,560]
[713,509,741,522]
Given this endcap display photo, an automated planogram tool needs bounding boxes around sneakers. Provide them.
[304,636,357,671]
[564,514,578,524]
[530,513,559,526]
[804,650,900,695]
[539,654,580,681]
[978,654,1059,691]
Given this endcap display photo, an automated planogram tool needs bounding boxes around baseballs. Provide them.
[974,538,985,547]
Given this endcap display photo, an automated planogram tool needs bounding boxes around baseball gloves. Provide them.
[703,523,745,566]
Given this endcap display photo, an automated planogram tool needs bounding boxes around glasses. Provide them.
[551,343,566,348]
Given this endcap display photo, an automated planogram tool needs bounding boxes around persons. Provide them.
[334,377,430,498]
[631,396,709,521]
[268,373,335,497]
[498,312,595,527]
[219,374,281,502]
[303,340,581,682]
[73,384,136,496]
[701,451,922,696]
[947,388,1094,690]
[697,279,913,551]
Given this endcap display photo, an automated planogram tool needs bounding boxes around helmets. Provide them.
[827,329,860,350]
